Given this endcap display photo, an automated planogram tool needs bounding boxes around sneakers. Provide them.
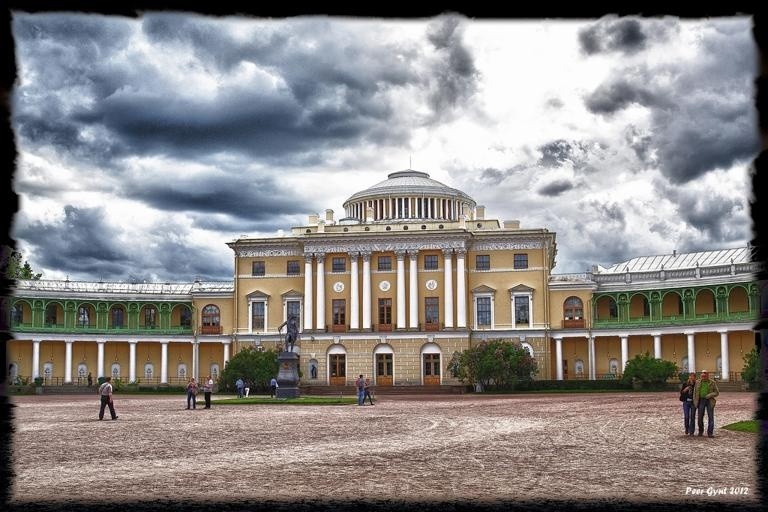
[112,415,119,420]
[690,431,694,437]
[185,406,211,410]
[698,431,703,436]
[99,418,103,420]
[684,429,689,434]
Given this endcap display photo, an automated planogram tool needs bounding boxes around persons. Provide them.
[361,378,375,406]
[200,374,214,409]
[183,377,198,410]
[243,380,251,398]
[277,313,300,352]
[269,376,278,398]
[357,374,365,406]
[86,371,93,386]
[235,377,245,399]
[692,369,720,439]
[97,377,118,422]
[678,372,698,437]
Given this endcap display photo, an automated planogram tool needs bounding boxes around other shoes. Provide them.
[708,432,714,438]
